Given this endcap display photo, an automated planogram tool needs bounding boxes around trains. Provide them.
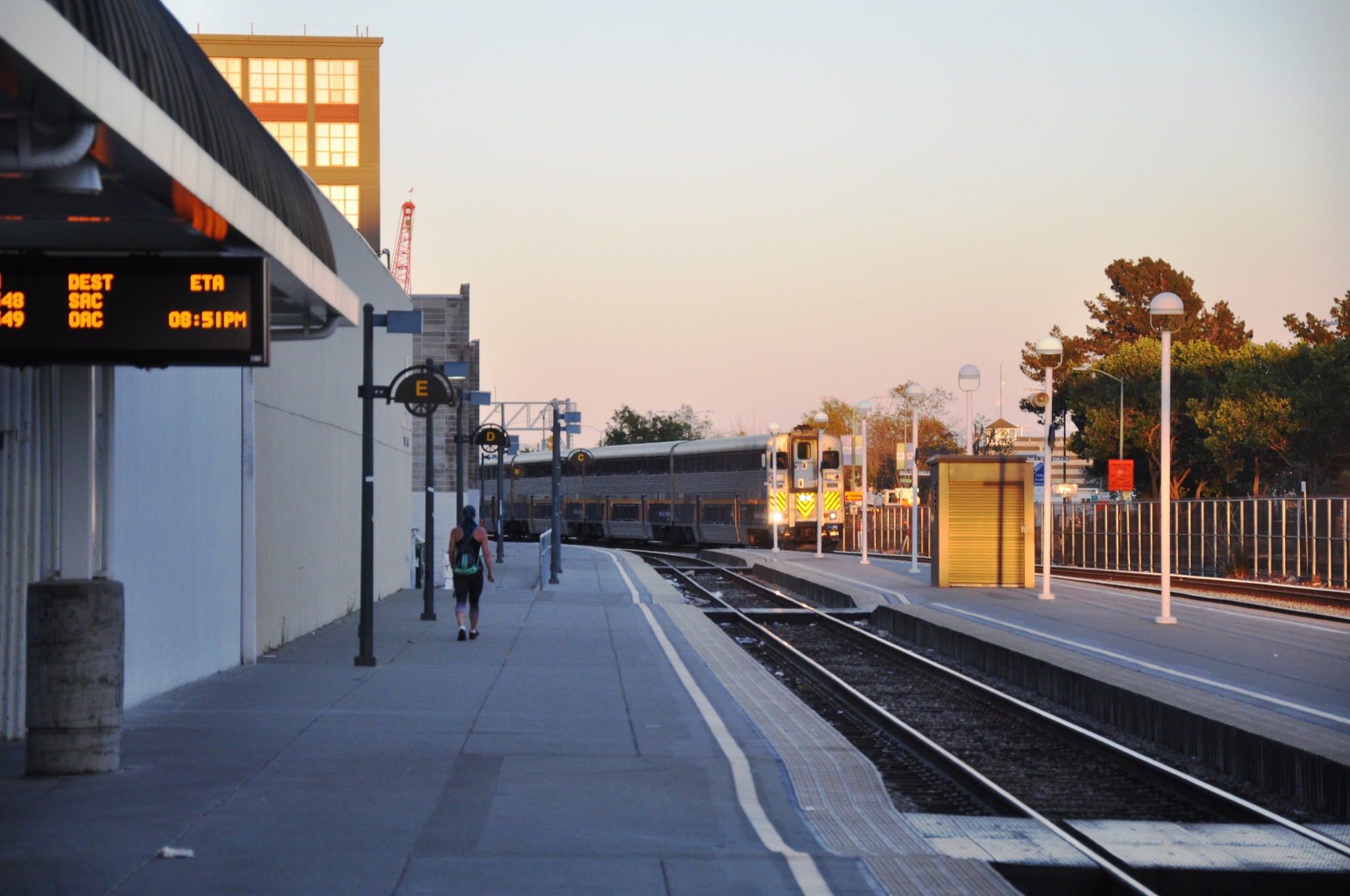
[477,424,846,551]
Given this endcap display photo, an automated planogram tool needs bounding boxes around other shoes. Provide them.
[457,629,466,641]
[469,630,479,639]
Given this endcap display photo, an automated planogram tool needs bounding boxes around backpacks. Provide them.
[452,525,482,574]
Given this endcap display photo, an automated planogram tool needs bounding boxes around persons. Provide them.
[449,505,496,641]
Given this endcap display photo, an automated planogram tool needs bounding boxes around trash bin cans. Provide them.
[28,569,128,773]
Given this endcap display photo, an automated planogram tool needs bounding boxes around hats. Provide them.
[464,505,475,515]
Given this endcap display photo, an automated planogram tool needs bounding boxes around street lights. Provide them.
[1149,292,1186,624]
[766,422,781,551]
[957,363,980,455]
[813,409,830,559]
[1035,335,1064,601]
[855,399,873,565]
[480,452,497,527]
[905,381,926,573]
[1072,367,1124,503]
